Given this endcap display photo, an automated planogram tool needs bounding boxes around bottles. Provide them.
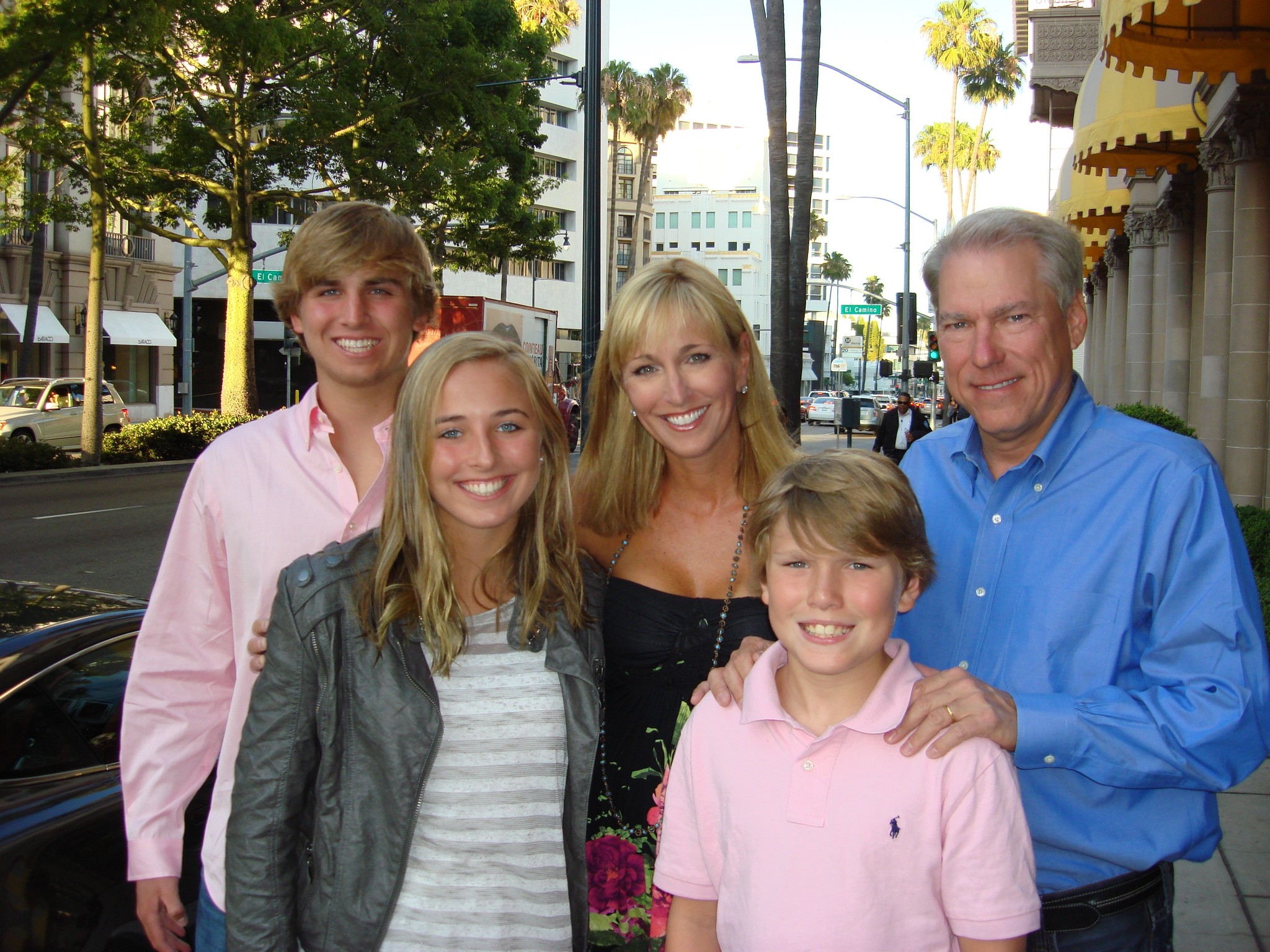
[905,428,912,442]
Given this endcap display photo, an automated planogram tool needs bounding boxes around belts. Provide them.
[1039,867,1166,933]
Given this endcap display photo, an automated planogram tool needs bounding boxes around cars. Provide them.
[0,578,217,952]
[800,390,944,436]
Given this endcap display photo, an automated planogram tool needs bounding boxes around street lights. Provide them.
[738,54,910,392]
[833,194,938,244]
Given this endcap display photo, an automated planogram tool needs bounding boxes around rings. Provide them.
[943,705,957,726]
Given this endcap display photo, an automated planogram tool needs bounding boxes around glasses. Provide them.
[898,401,908,405]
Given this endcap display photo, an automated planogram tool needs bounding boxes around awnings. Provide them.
[1081,257,1100,279]
[1074,229,1110,257]
[1059,189,1131,235]
[1072,102,1208,177]
[0,303,71,343]
[1097,1,1269,87]
[101,309,177,347]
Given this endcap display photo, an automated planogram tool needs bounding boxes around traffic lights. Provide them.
[896,385,899,391]
[927,331,942,362]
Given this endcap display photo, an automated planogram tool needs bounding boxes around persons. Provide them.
[957,405,970,422]
[687,206,1270,952]
[651,449,1046,952]
[117,200,434,951]
[216,331,600,950]
[948,395,958,424]
[248,251,809,952]
[872,392,926,465]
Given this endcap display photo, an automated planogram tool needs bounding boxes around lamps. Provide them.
[75,306,87,335]
[165,312,178,333]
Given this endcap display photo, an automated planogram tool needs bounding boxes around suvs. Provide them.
[0,378,131,452]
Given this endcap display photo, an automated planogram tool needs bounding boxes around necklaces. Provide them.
[602,502,750,837]
